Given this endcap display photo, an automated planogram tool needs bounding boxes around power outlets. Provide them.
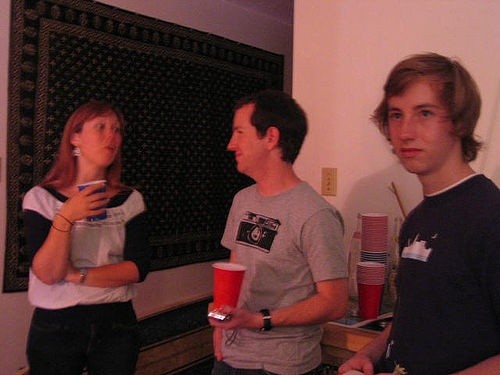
[321,168,337,197]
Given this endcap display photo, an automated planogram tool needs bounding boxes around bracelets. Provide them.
[56,213,74,225]
[52,224,72,232]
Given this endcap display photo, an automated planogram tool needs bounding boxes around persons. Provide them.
[207,90,349,375]
[22,101,151,375]
[338,51,500,375]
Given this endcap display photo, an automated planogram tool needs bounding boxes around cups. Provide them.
[75,179,107,223]
[356,213,389,320]
[210,262,247,314]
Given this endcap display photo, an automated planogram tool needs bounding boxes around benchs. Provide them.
[81,293,218,375]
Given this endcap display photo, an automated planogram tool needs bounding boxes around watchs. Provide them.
[259,309,272,332]
[79,267,87,284]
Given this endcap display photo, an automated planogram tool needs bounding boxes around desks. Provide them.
[319,295,394,375]
